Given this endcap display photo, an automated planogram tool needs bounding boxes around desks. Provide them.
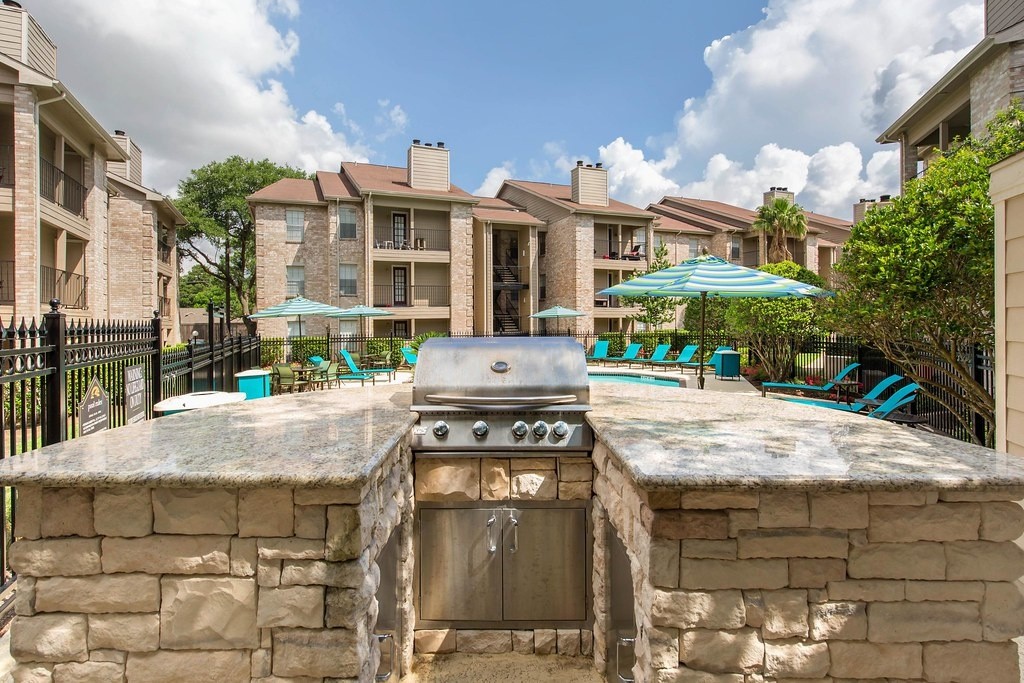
[671,354,680,368]
[854,398,886,412]
[360,356,371,374]
[612,352,624,363]
[291,367,323,393]
[828,379,861,404]
[416,237,428,251]
[692,356,707,369]
[383,241,394,250]
[640,353,652,366]
[872,411,929,428]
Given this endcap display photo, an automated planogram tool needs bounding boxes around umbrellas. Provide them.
[526,303,587,336]
[595,247,838,389]
[246,294,396,369]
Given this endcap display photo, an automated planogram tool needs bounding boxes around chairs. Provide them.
[399,240,413,250]
[628,344,673,370]
[585,341,610,366]
[399,347,418,364]
[603,344,643,368]
[621,245,641,260]
[762,362,862,398]
[250,349,396,396]
[681,346,733,376]
[651,345,700,371]
[866,382,920,417]
[879,394,916,420]
[774,374,905,414]
[375,239,386,250]
